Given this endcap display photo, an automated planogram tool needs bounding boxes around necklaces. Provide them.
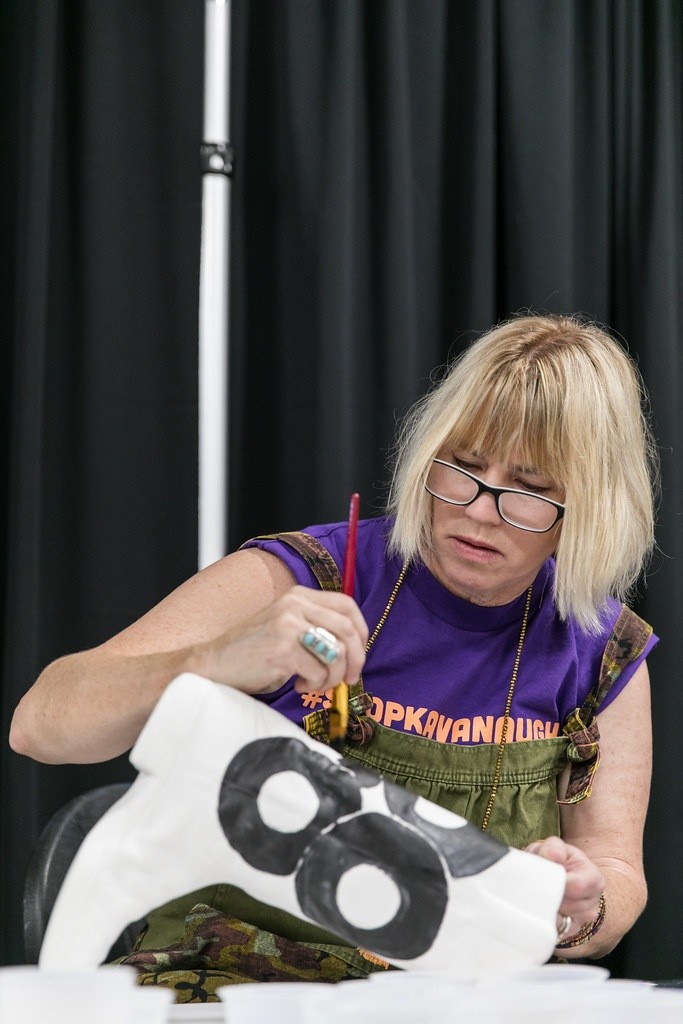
[353,555,533,833]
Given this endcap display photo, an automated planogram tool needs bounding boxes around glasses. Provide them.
[421,458,566,534]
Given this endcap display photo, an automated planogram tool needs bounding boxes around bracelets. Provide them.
[552,893,606,949]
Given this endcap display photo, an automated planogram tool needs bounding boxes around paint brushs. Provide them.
[328,492,360,755]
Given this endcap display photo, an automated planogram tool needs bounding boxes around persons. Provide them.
[6,311,658,968]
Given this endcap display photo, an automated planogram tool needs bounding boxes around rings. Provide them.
[301,627,342,663]
[557,916,572,938]
